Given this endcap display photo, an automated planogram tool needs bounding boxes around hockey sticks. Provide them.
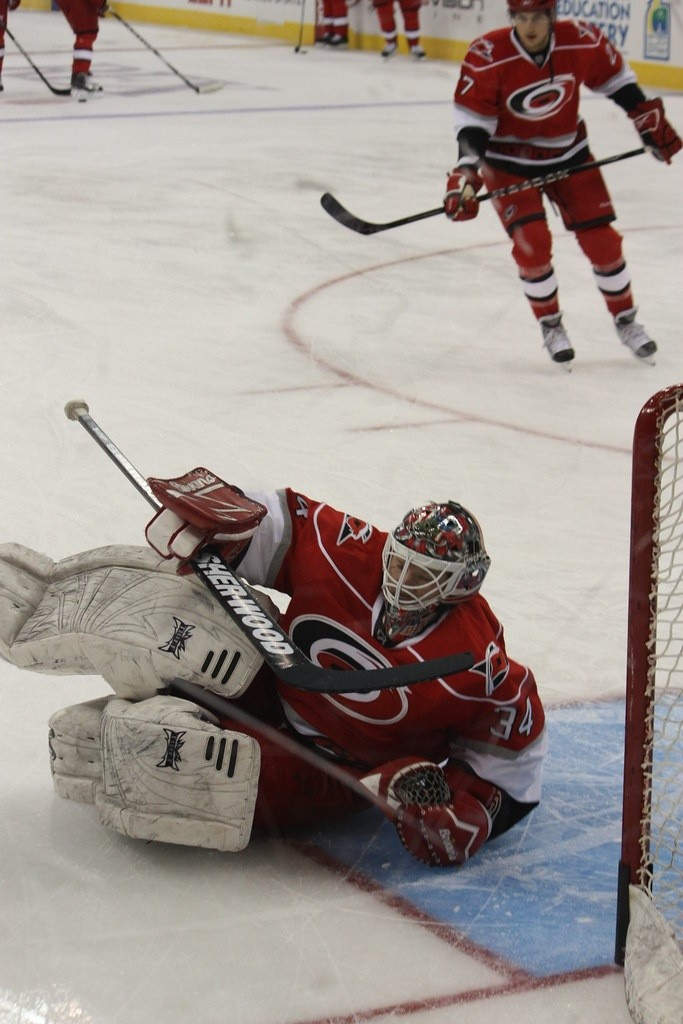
[109,9,225,95]
[294,1,310,56]
[64,397,475,695]
[320,145,654,235]
[1,21,73,97]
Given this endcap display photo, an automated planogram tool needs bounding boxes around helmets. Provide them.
[381,501,490,640]
[506,0,557,18]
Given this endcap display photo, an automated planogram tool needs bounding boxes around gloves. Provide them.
[444,165,484,222]
[627,97,682,164]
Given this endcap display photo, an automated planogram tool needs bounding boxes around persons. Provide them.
[444,0,683,373]
[0,1,28,93]
[54,0,109,102]
[1,466,549,868]
[314,0,351,51]
[373,0,426,63]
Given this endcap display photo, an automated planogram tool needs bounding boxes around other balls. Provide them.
[78,98,87,102]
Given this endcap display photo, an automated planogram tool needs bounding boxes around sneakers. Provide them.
[315,32,333,49]
[325,33,351,49]
[71,70,104,103]
[382,41,399,62]
[614,304,658,366]
[540,311,575,372]
[409,45,427,62]
